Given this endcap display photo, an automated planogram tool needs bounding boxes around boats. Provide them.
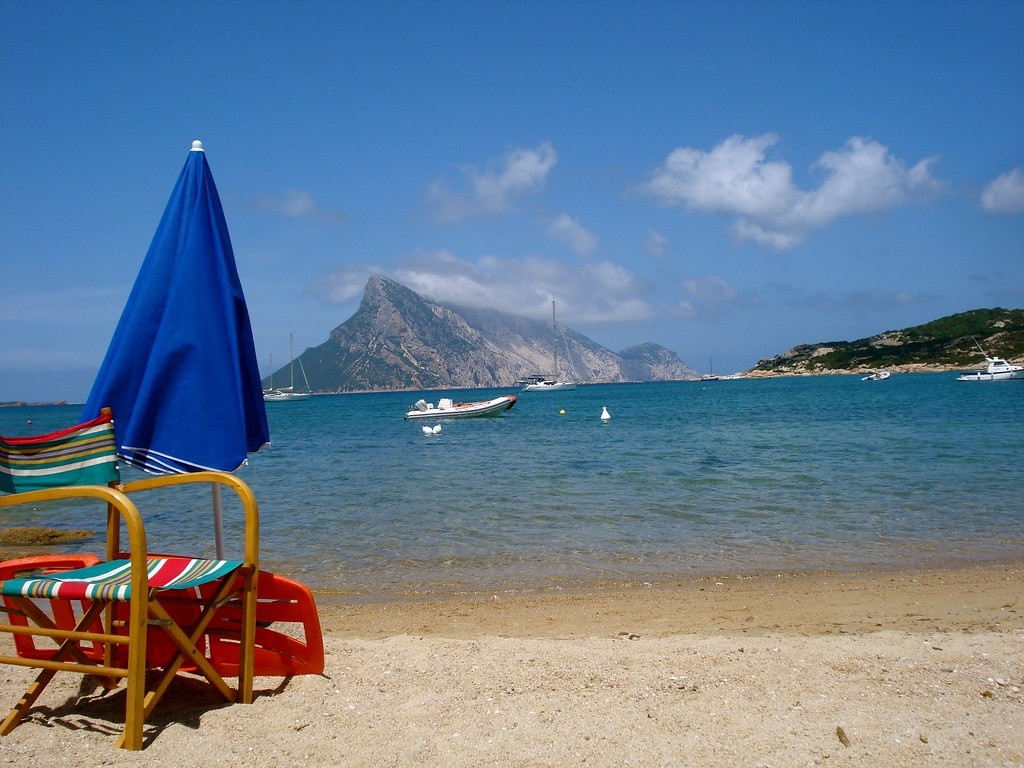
[955,356,1024,381]
[700,351,719,381]
[404,395,517,421]
[860,371,892,382]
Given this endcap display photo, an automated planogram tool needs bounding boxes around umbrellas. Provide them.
[79,139,270,562]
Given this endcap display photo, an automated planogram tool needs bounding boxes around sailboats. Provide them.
[259,332,313,402]
[522,299,580,392]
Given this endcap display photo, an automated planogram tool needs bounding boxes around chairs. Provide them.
[0,407,260,752]
